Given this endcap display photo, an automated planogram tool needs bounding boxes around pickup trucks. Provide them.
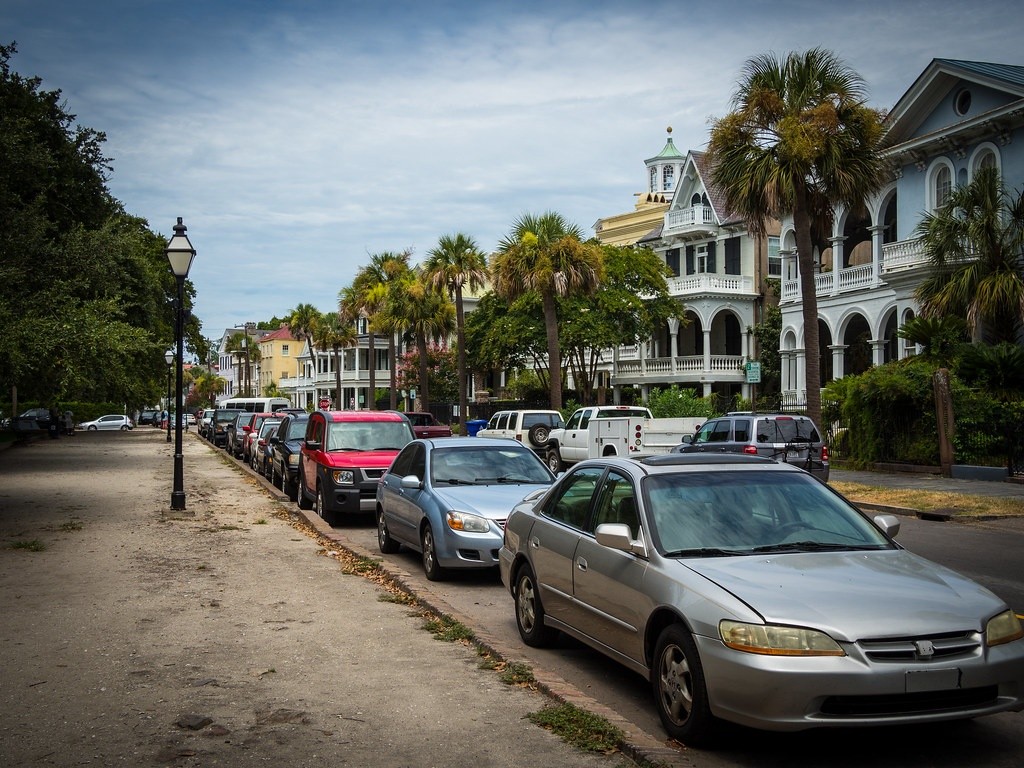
[401,413,454,440]
[544,406,708,477]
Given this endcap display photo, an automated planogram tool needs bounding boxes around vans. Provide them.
[219,398,290,413]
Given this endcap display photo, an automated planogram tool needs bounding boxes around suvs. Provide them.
[476,409,564,450]
[271,412,313,501]
[669,411,830,483]
[208,409,248,447]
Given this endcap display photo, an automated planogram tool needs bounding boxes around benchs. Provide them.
[554,497,619,533]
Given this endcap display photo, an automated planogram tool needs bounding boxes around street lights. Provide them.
[164,217,197,510]
[164,348,174,442]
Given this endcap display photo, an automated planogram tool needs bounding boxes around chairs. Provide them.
[617,497,656,540]
[711,488,763,547]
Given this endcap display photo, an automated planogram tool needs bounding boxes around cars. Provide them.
[296,410,419,526]
[243,408,305,472]
[257,429,278,481]
[2,409,74,431]
[499,452,1023,745]
[226,413,254,459]
[197,409,214,438]
[79,414,134,430]
[138,409,188,430]
[183,414,197,425]
[376,436,559,581]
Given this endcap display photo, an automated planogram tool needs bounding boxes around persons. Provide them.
[37,403,74,436]
[162,408,168,429]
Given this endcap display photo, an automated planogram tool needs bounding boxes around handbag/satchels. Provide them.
[161,411,165,419]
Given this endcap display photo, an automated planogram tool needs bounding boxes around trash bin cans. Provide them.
[466,420,488,437]
[48,422,59,439]
